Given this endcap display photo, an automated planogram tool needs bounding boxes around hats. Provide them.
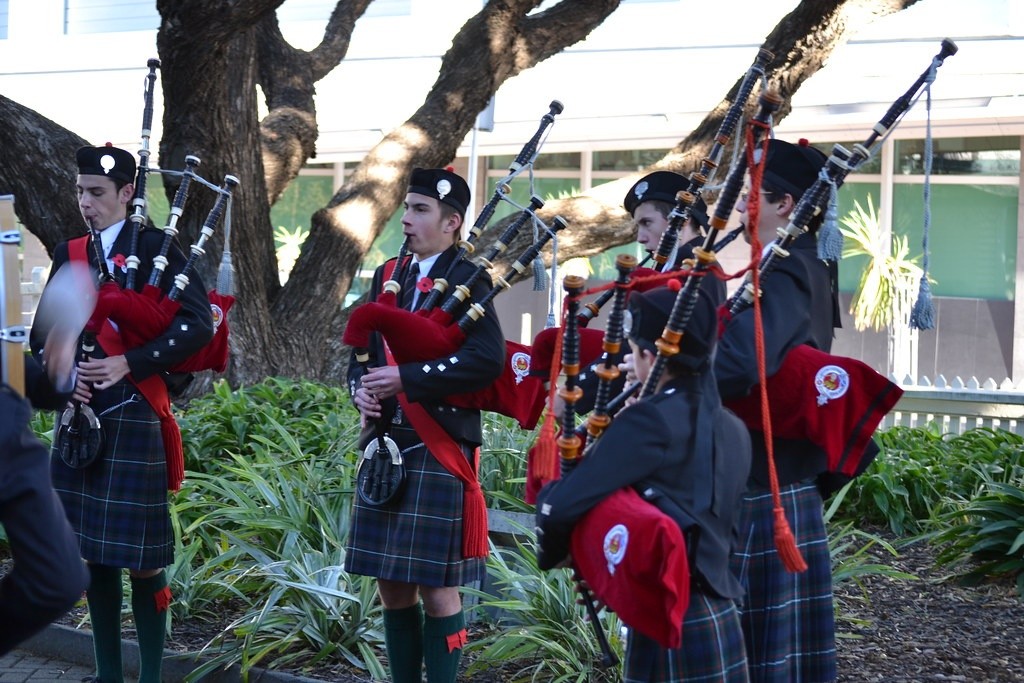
[623,170,708,226]
[619,285,721,367]
[408,165,471,216]
[77,141,137,184]
[746,133,830,203]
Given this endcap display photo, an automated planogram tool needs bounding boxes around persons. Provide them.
[534,139,840,683]
[0,381,91,655]
[347,168,508,683]
[28,147,214,683]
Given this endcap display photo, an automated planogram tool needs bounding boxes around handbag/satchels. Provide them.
[56,401,106,468]
[354,434,410,508]
